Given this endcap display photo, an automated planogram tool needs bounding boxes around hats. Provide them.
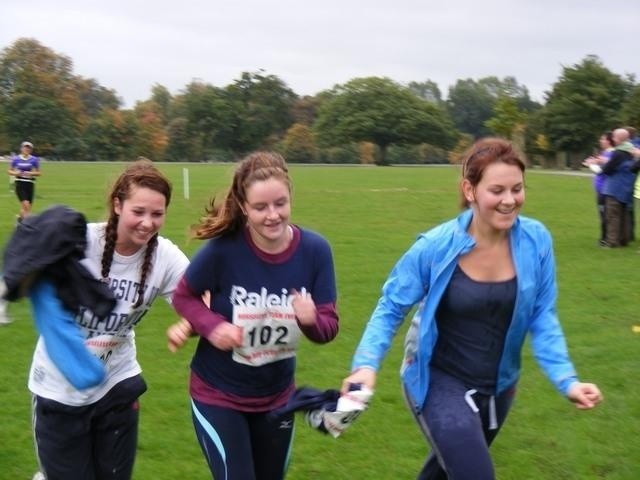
[21,141,33,148]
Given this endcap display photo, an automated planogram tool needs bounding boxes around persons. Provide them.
[7,142,41,228]
[26,161,211,480]
[172,149,339,480]
[584,126,640,247]
[341,136,604,480]
